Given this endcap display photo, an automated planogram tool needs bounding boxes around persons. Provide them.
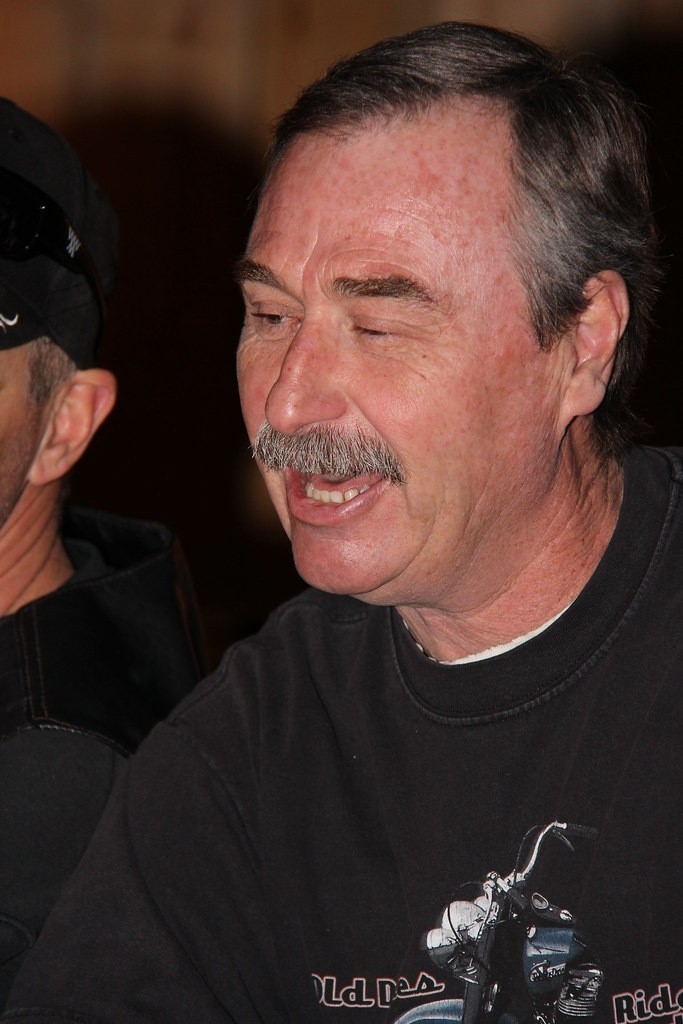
[0,85,117,1012]
[1,19,683,1024]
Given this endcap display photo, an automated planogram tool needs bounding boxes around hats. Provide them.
[1,98,118,370]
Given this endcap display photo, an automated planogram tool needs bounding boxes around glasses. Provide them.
[0,166,107,361]
